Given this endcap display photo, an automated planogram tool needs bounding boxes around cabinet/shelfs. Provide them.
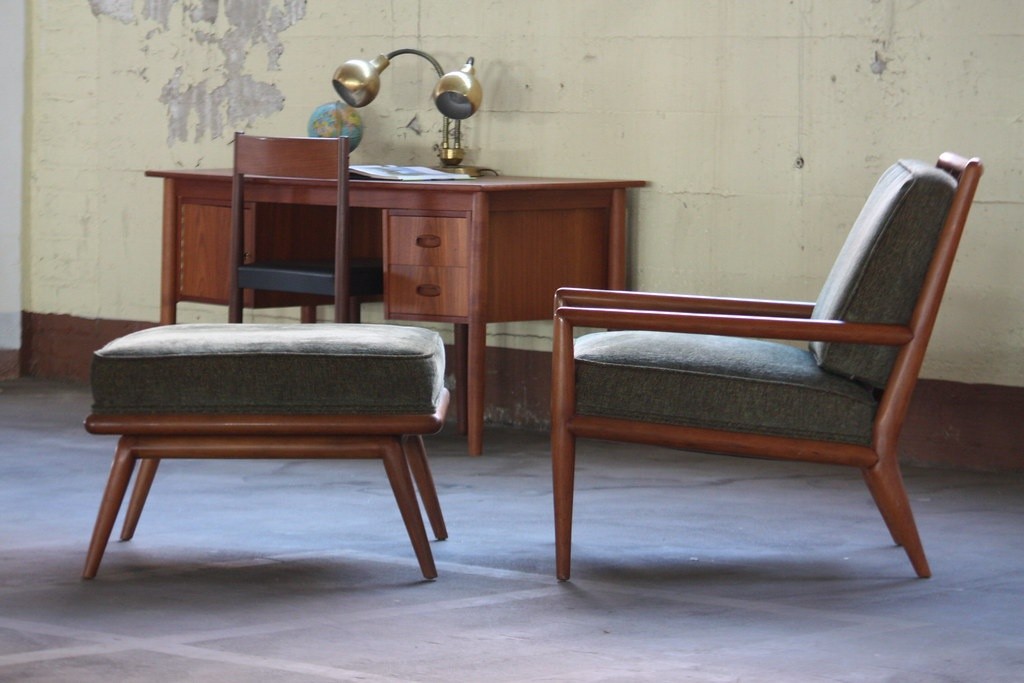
[145,169,646,456]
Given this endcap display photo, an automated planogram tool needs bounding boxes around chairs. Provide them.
[227,130,470,436]
[549,150,985,585]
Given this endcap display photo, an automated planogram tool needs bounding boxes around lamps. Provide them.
[332,47,485,177]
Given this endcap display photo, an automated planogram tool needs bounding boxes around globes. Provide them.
[307,100,364,155]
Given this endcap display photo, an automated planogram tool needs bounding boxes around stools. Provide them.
[80,324,451,580]
